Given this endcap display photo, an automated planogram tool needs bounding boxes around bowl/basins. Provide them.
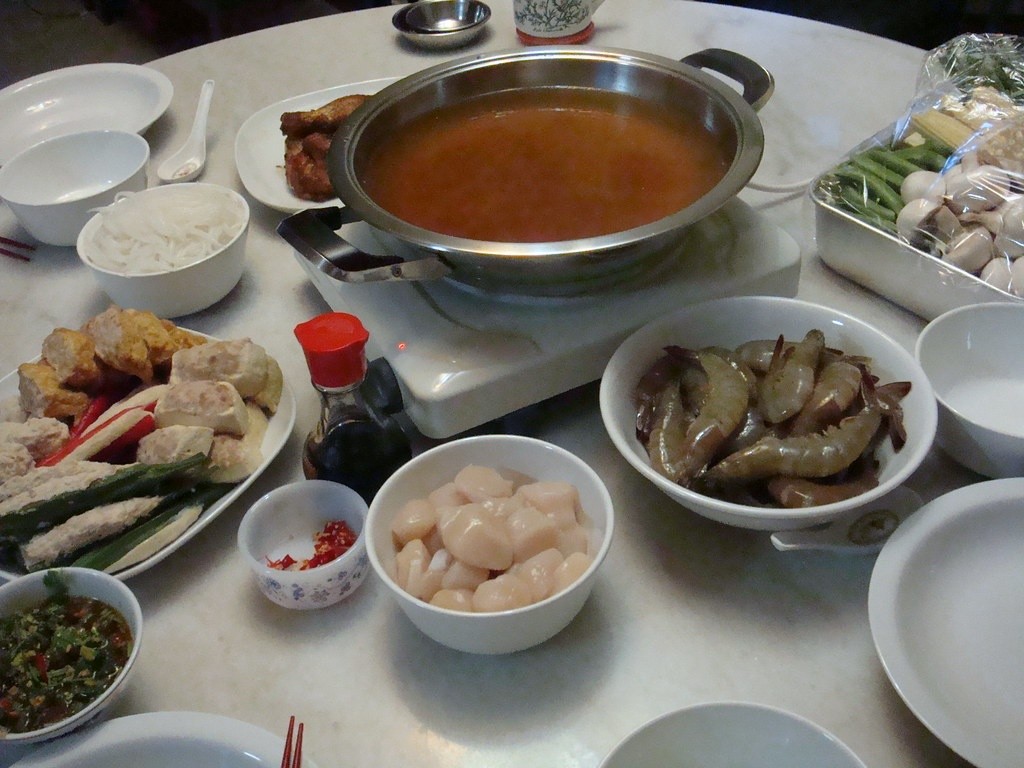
[598,295,1023,532]
[0,566,142,745]
[405,0,489,34]
[76,183,249,317]
[391,0,491,48]
[0,128,150,246]
[365,434,614,656]
[598,701,867,768]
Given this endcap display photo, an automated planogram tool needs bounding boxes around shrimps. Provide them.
[640,329,914,506]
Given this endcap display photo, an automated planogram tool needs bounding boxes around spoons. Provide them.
[768,484,923,554]
[155,79,215,184]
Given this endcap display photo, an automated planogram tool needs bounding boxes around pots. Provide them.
[276,43,776,298]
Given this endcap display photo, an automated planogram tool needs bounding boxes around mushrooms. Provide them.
[894,153,1024,300]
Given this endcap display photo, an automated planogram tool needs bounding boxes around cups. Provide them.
[237,480,367,611]
[512,0,607,38]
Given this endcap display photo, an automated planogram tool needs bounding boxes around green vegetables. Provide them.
[0,569,131,734]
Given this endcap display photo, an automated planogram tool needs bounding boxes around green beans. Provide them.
[830,140,958,228]
[0,452,236,578]
[935,35,1024,107]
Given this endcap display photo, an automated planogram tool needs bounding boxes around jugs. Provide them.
[292,312,414,507]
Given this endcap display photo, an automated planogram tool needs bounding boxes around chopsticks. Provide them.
[0,236,36,262]
[281,715,304,768]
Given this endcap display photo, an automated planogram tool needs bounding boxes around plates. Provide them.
[867,477,1024,768]
[233,76,407,212]
[6,711,319,768]
[0,63,174,167]
[0,325,295,581]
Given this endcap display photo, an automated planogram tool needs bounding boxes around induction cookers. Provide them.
[279,197,802,443]
